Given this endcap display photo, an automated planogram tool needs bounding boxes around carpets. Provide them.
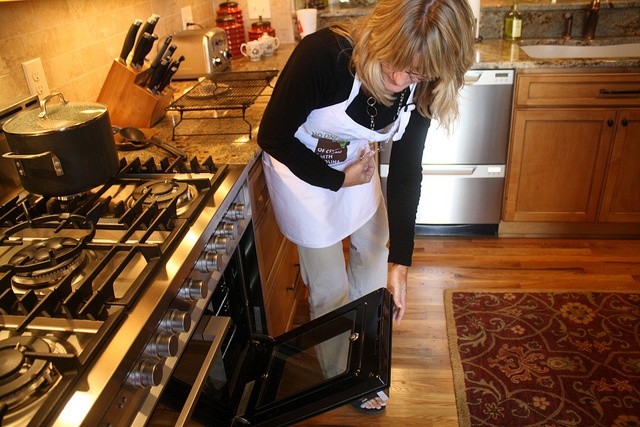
[442,284,639,426]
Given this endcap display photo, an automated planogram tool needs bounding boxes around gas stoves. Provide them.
[0,95,251,427]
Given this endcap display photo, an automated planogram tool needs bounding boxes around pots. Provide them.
[2,92,122,197]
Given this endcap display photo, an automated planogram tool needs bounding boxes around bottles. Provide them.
[214,0,245,58]
[249,16,275,40]
[215,18,242,59]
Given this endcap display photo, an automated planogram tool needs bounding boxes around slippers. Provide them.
[349,393,386,415]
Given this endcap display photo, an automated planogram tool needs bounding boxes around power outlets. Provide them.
[180,5,196,31]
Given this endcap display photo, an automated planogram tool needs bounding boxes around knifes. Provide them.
[146,59,168,94]
[145,33,159,54]
[160,59,179,84]
[152,14,160,21]
[149,34,173,67]
[118,18,142,67]
[130,32,150,68]
[154,44,177,74]
[154,56,172,89]
[134,17,157,54]
[176,55,185,68]
[156,66,178,95]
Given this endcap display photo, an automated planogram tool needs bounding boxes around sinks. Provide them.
[518,39,640,61]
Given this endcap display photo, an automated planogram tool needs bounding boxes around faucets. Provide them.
[584,0,600,39]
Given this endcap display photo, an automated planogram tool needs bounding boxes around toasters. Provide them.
[158,28,233,79]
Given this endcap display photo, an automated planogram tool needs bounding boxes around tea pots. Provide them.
[257,32,279,56]
[241,40,264,62]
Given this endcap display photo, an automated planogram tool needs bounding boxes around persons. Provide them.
[257,0,476,415]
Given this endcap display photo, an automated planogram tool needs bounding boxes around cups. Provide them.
[296,8,318,40]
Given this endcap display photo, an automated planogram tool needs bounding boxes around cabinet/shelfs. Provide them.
[245,151,304,337]
[497,70,639,241]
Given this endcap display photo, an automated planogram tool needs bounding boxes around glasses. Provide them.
[403,67,431,84]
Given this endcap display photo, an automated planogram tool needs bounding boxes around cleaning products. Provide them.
[467,0,480,38]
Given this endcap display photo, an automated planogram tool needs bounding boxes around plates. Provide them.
[184,83,228,98]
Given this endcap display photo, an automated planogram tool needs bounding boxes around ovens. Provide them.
[126,166,394,427]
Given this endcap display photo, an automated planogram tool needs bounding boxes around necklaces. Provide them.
[358,89,407,160]
